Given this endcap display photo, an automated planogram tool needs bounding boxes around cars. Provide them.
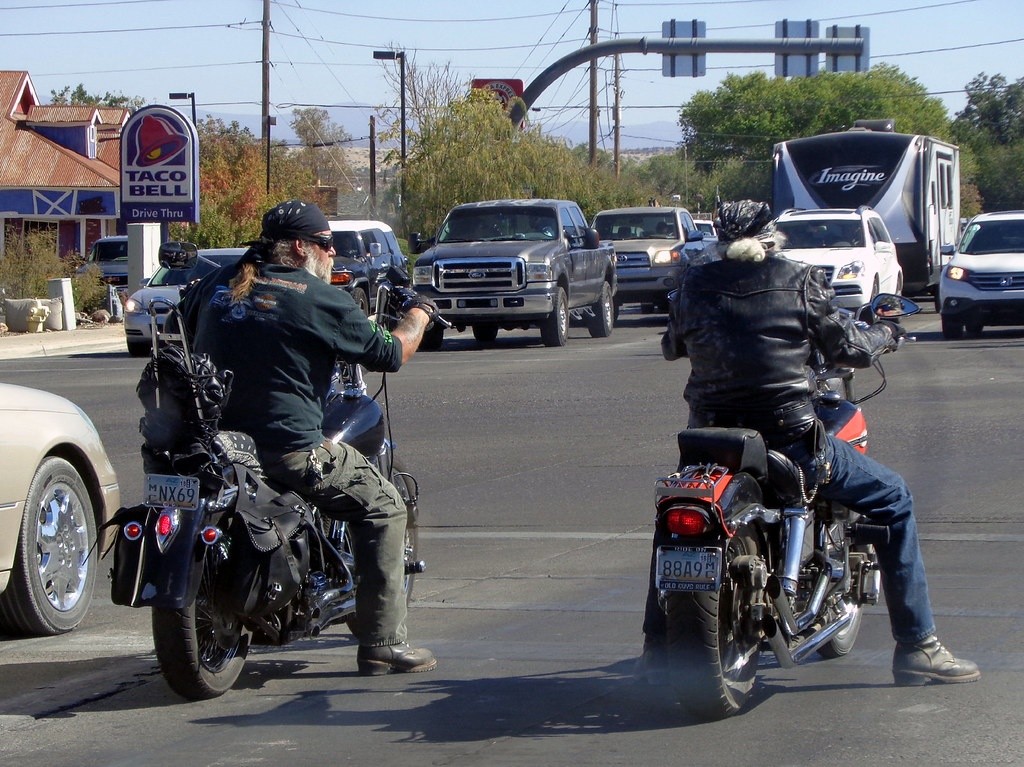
[76,236,128,293]
[328,220,408,317]
[124,248,250,357]
[0,383,120,637]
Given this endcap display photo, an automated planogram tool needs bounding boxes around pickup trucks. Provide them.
[409,199,619,352]
[590,207,718,322]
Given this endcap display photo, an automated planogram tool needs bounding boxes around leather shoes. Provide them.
[891,633,981,686]
[356,641,437,677]
[632,641,670,686]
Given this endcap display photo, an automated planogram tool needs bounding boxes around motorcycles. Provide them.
[654,288,925,721]
[82,242,453,704]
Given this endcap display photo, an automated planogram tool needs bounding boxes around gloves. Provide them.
[406,293,440,324]
[872,320,906,340]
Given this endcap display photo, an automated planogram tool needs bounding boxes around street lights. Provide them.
[169,93,196,129]
[373,51,405,206]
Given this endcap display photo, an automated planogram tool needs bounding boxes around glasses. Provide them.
[875,301,905,311]
[304,233,334,253]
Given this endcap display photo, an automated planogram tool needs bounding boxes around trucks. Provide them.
[772,120,961,313]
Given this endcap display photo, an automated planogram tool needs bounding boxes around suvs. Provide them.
[938,210,1024,340]
[773,205,904,318]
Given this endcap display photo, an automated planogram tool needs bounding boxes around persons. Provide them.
[633,199,982,688]
[460,220,491,240]
[876,295,904,318]
[655,221,674,236]
[163,196,435,674]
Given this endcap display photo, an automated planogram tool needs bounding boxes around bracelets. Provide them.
[408,303,433,325]
